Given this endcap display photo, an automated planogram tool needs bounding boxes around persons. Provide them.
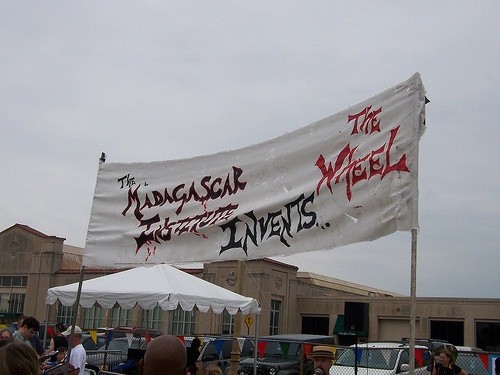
[0,315,223,375]
[310,345,339,375]
[431,350,468,375]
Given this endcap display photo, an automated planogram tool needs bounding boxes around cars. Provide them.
[0,318,255,375]
[328,339,488,375]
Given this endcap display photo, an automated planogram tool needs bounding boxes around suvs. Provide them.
[237,333,338,375]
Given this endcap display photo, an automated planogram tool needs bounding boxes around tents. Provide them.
[42,263,262,375]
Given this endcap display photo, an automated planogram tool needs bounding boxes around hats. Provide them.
[61,325,82,335]
[309,346,340,360]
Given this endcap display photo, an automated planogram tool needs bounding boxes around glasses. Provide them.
[29,329,38,335]
